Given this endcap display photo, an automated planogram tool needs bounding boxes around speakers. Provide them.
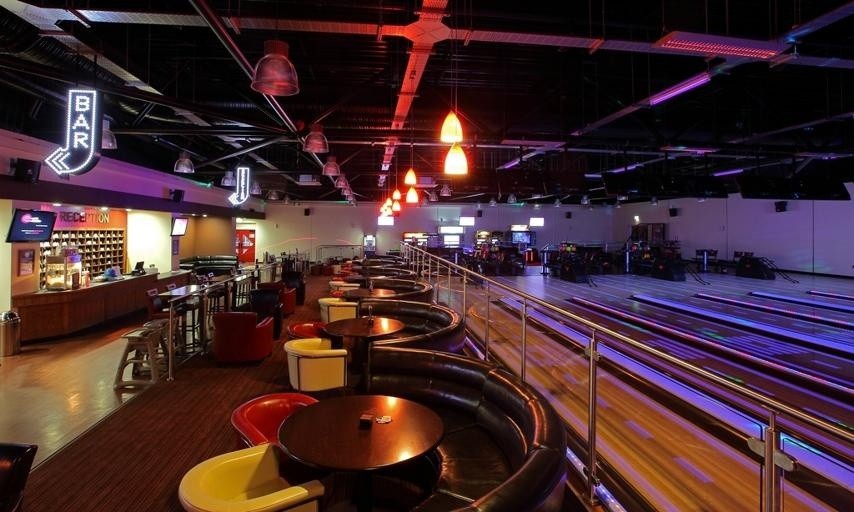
[304,208,310,216]
[477,210,482,217]
[669,207,677,217]
[775,201,787,212]
[566,212,571,218]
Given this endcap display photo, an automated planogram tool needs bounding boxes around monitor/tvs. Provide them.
[135,262,144,269]
[169,216,189,236]
[6,208,57,243]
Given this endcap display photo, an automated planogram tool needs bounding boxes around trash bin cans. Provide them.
[0,311,22,357]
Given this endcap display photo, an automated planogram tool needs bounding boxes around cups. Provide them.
[39,231,123,289]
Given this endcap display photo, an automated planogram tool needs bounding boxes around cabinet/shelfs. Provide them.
[38,227,124,291]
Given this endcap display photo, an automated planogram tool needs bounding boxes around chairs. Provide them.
[1,440,40,511]
